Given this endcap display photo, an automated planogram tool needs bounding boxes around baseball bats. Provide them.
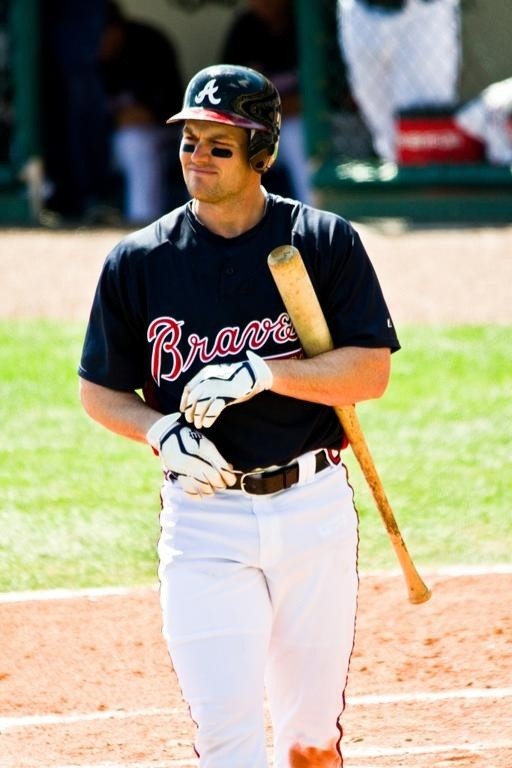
[266,245,431,606]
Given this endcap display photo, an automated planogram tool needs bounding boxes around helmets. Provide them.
[167,64,282,173]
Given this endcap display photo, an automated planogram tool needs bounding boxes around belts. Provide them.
[227,451,329,494]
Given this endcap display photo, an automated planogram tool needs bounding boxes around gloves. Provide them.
[180,350,273,429]
[146,412,236,499]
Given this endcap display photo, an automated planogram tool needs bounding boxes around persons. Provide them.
[220,0,360,208]
[78,62,403,768]
[17,0,186,228]
